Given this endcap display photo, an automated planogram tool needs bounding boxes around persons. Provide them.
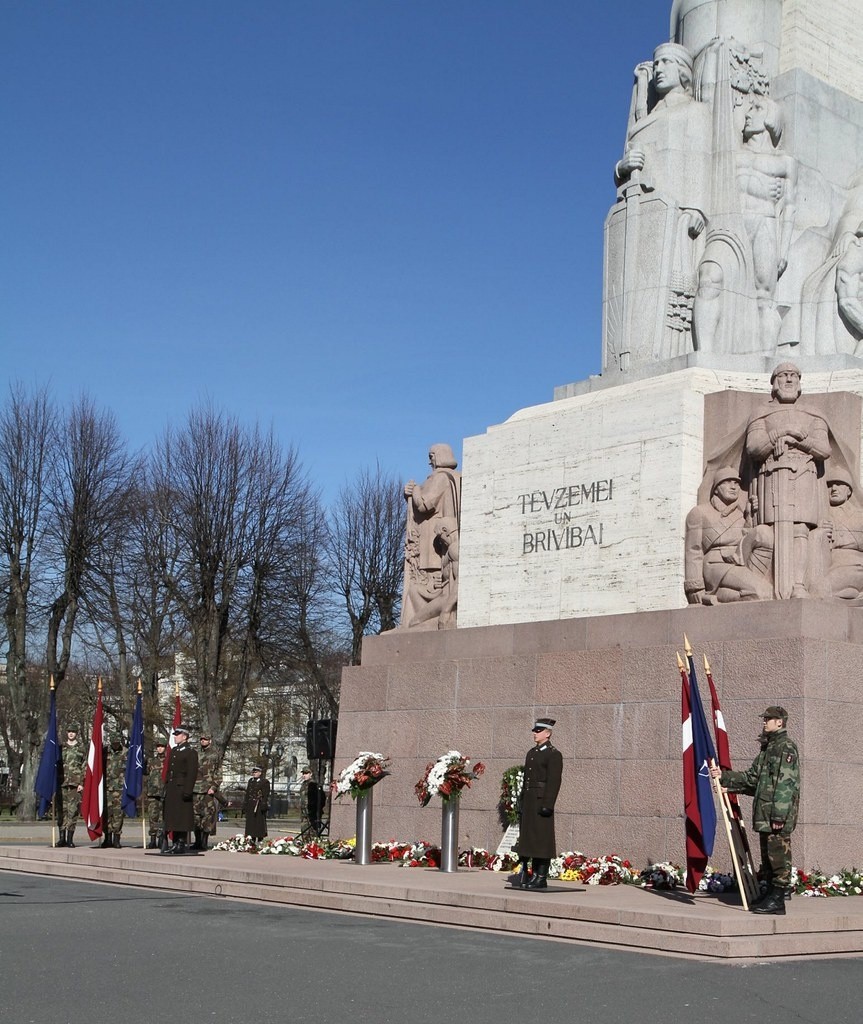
[55,724,88,848]
[614,35,863,355]
[400,442,460,630]
[710,705,801,915]
[146,726,224,854]
[242,765,270,842]
[684,361,863,606]
[300,766,316,844]
[103,732,129,848]
[518,717,564,888]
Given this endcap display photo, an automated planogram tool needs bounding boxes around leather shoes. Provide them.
[748,890,786,915]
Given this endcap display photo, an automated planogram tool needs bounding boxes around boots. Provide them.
[112,833,122,849]
[163,831,187,854]
[190,829,210,850]
[55,830,67,847]
[158,836,164,848]
[146,835,157,849]
[65,830,75,848]
[100,834,112,847]
[522,872,548,889]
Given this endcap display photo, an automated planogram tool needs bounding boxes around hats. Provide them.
[210,786,217,792]
[66,726,79,732]
[172,724,192,736]
[300,766,312,773]
[199,731,212,740]
[251,767,263,772]
[531,718,556,733]
[759,706,789,718]
[154,739,168,747]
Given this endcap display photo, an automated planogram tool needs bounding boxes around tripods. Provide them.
[294,759,329,840]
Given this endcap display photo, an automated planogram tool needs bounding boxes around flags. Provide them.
[33,690,61,818]
[121,694,144,818]
[162,697,181,838]
[81,695,104,841]
[681,656,749,895]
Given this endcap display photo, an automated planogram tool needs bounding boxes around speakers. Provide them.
[306,719,337,759]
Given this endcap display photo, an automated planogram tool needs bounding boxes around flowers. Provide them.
[495,765,526,827]
[330,751,398,795]
[213,833,863,898]
[414,750,482,808]
